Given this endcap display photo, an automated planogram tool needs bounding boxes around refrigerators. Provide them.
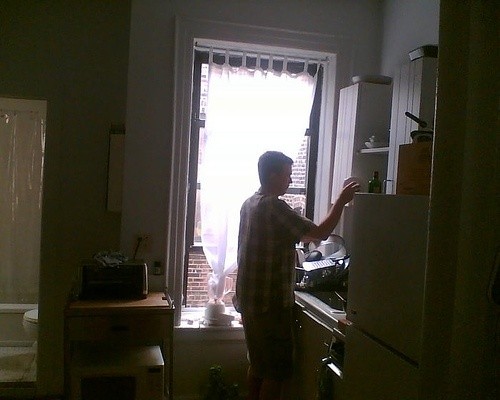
[342,191,431,400]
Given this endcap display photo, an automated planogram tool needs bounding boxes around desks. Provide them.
[63,290,176,400]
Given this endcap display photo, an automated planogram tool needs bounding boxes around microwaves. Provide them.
[71,346,165,399]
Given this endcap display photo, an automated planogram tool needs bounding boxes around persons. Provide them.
[232,151,360,400]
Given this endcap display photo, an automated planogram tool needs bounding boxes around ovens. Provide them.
[79,260,149,301]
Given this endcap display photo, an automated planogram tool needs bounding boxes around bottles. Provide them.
[368,171,382,194]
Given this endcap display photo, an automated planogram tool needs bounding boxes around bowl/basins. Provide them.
[369,135,385,143]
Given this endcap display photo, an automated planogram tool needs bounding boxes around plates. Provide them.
[365,141,387,148]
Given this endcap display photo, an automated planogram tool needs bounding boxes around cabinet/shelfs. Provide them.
[329,55,437,204]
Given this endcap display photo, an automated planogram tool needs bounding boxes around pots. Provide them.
[405,110,434,143]
